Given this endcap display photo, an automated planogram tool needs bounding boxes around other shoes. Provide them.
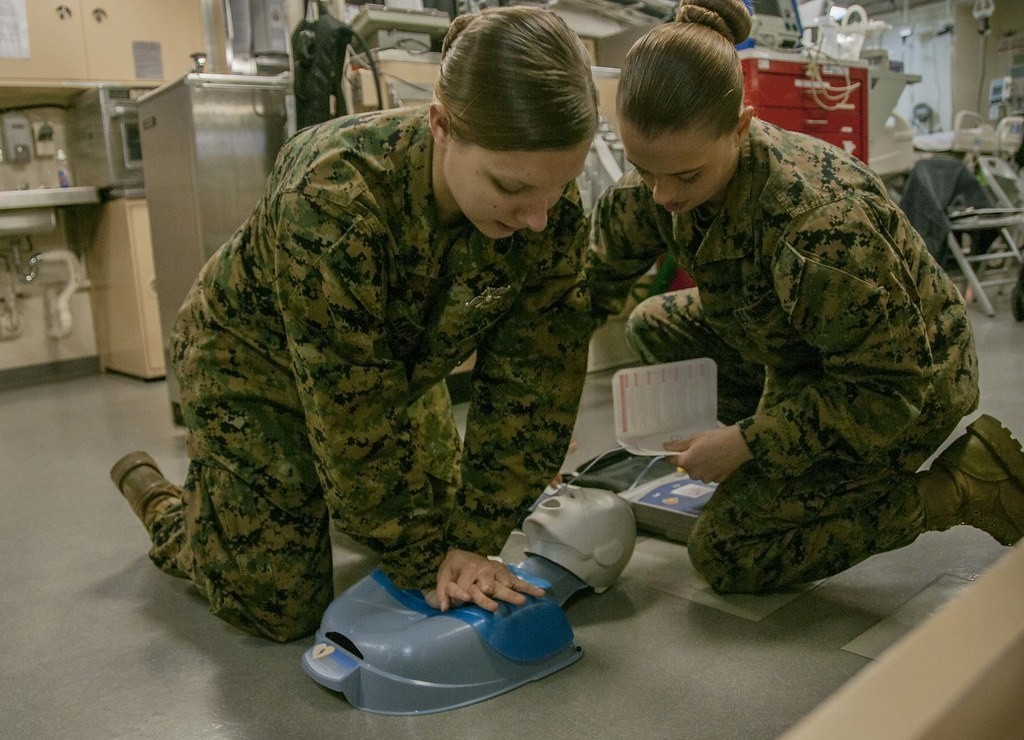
[916,414,1024,548]
[110,450,182,535]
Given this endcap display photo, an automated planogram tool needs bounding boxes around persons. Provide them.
[304,487,636,714]
[109,6,592,645]
[589,0,1024,595]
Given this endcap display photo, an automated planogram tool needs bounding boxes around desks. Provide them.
[912,128,980,151]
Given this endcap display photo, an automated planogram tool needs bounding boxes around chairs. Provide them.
[904,110,1023,319]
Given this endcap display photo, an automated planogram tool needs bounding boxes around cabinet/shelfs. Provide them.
[740,55,869,171]
[134,74,306,423]
[0,0,232,382]
